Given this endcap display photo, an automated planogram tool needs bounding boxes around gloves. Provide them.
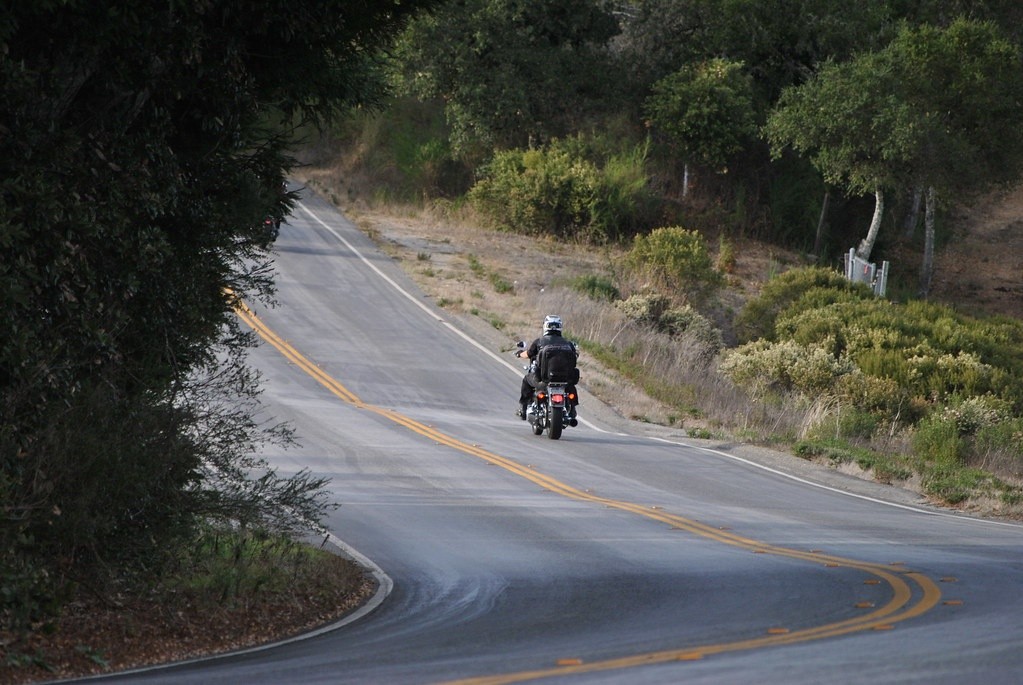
[515,350,523,358]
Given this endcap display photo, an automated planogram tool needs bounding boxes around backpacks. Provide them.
[534,337,579,383]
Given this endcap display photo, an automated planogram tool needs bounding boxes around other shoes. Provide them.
[568,409,577,417]
[518,408,526,420]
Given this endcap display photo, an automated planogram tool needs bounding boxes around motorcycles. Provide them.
[508,341,579,439]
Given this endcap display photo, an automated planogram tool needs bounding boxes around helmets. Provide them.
[543,315,563,335]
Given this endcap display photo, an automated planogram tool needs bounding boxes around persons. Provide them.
[514,315,577,421]
